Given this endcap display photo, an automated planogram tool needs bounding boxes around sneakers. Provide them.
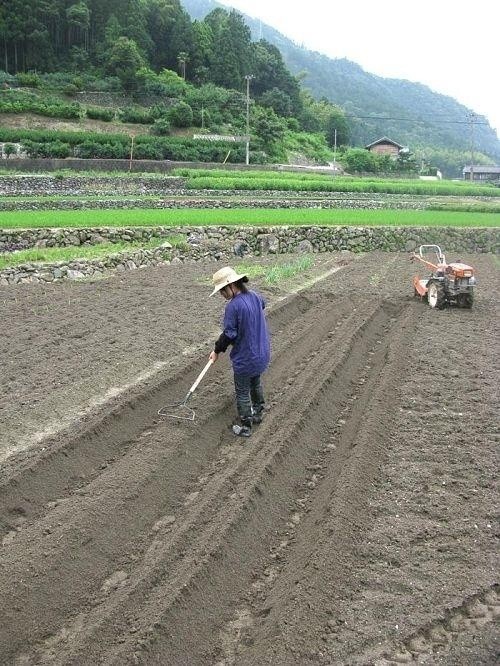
[252,412,264,423]
[232,424,251,437]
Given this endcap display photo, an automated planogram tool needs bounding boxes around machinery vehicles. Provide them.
[409,244,477,310]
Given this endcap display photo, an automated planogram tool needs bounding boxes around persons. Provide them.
[209,266,271,436]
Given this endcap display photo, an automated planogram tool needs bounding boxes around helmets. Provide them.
[209,266,246,297]
